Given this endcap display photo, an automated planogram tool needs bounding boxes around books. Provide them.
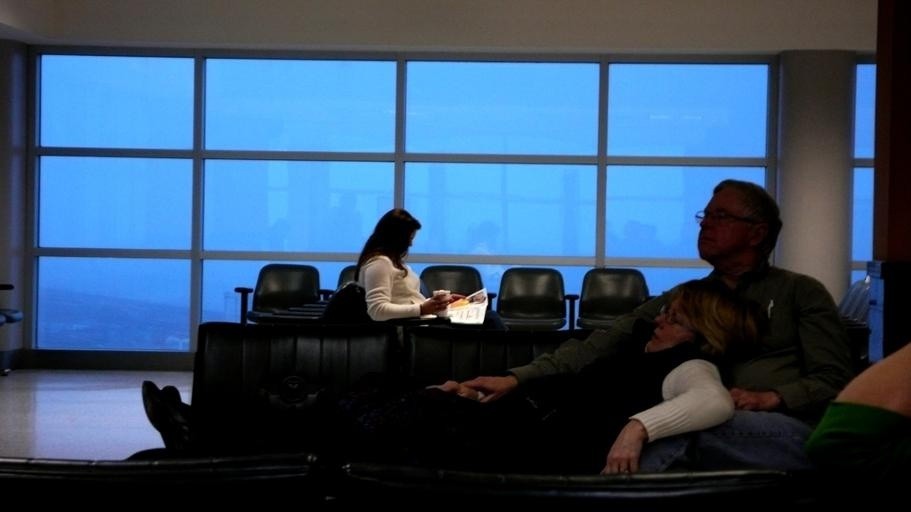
[435,288,490,324]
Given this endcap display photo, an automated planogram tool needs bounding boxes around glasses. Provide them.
[660,305,697,334]
[696,211,749,224]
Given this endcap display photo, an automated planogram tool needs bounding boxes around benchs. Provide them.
[191,322,592,406]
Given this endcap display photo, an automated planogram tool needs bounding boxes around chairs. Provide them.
[344,282,450,327]
[337,266,358,289]
[497,268,579,330]
[344,464,792,512]
[420,266,496,311]
[0,284,23,376]
[236,264,335,323]
[577,268,654,329]
[0,452,318,511]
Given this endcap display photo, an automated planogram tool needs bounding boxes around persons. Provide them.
[354,207,508,341]
[804,341,911,461]
[397,281,771,485]
[143,182,852,461]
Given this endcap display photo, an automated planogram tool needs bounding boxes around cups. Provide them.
[432,290,450,319]
